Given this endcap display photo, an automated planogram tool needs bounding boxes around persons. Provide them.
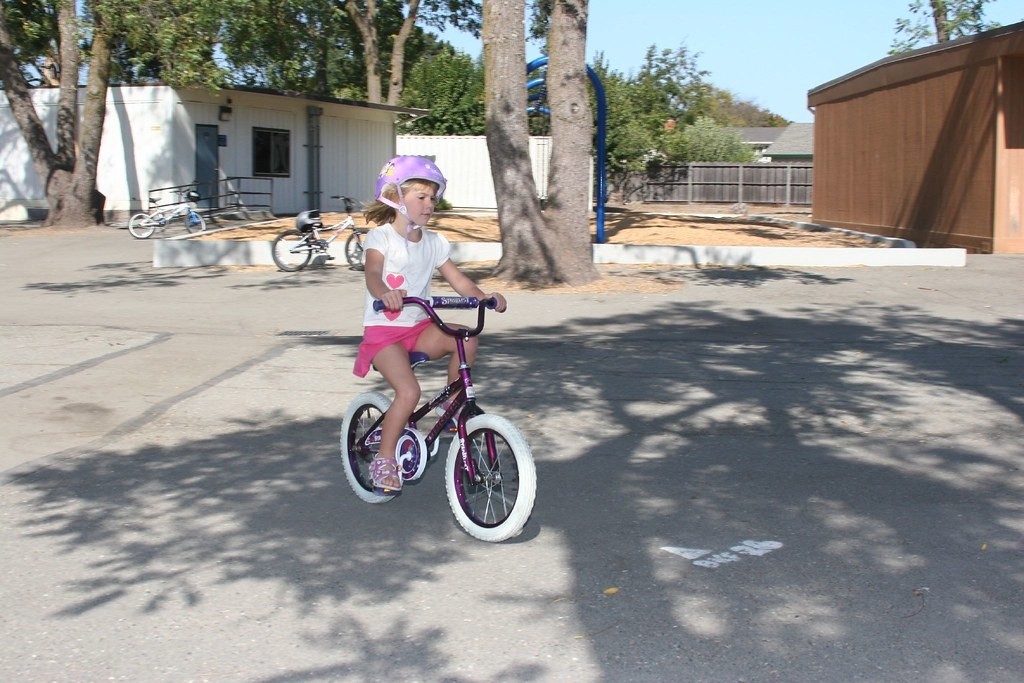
[353,156,507,490]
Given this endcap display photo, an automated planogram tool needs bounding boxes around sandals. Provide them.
[435,399,467,428]
[368,453,402,491]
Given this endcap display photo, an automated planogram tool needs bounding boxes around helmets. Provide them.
[296,209,323,232]
[374,156,446,201]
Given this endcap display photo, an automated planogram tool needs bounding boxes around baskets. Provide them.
[187,190,200,202]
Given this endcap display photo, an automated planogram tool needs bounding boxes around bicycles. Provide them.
[272,195,374,272]
[128,188,207,240]
[337,294,538,543]
